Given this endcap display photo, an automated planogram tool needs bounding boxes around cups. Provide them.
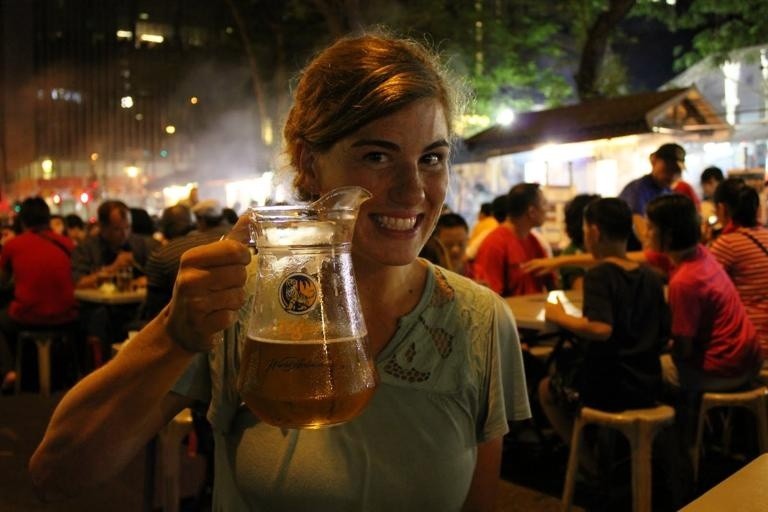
[204,183,378,432]
[116,266,134,296]
[93,266,112,292]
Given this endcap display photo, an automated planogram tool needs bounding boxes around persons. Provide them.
[517,192,763,480]
[179,187,200,210]
[144,205,214,299]
[0,196,76,393]
[708,180,768,375]
[537,199,671,479]
[193,201,232,243]
[649,155,701,217]
[470,202,492,239]
[27,27,531,512]
[441,205,451,216]
[436,215,470,274]
[71,200,156,370]
[618,144,686,253]
[466,195,511,265]
[559,192,601,286]
[700,167,723,200]
[470,183,560,296]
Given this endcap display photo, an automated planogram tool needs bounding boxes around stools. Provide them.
[562,401,677,512]
[156,406,196,512]
[19,314,55,392]
[691,364,767,484]
